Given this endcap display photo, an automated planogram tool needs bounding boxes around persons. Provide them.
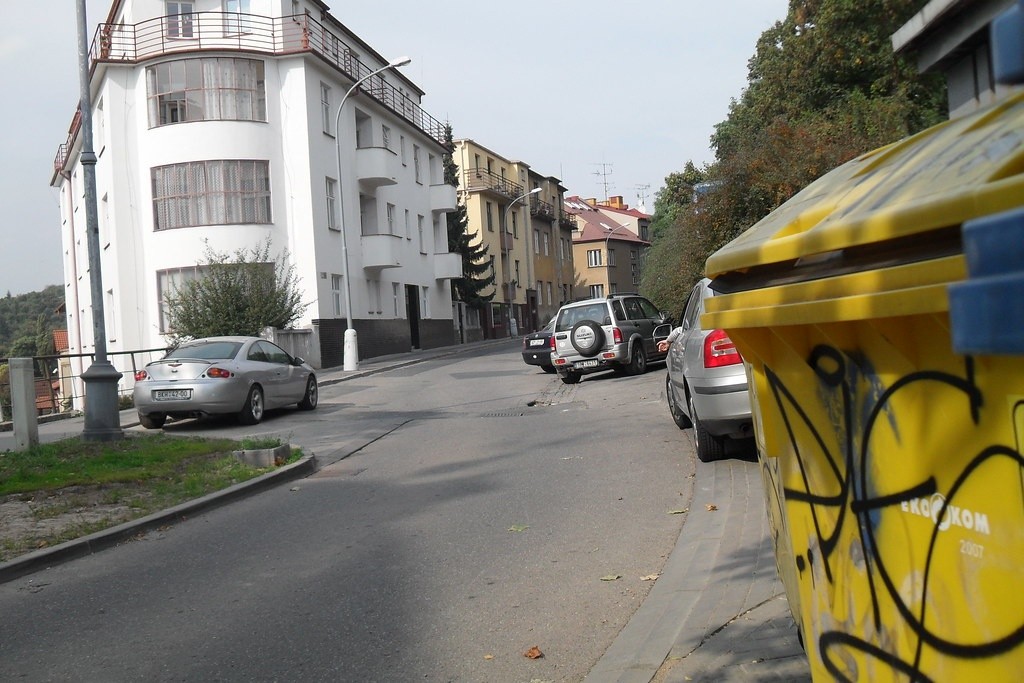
[656,327,682,352]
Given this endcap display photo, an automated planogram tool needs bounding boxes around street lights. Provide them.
[336,57,413,328]
[503,187,543,318]
[605,223,631,293]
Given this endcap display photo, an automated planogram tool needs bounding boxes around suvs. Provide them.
[549,292,673,385]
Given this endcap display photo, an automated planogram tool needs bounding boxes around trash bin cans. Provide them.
[702,92,1024,683]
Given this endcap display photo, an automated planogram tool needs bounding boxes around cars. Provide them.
[133,335,318,429]
[521,315,558,373]
[653,277,757,463]
